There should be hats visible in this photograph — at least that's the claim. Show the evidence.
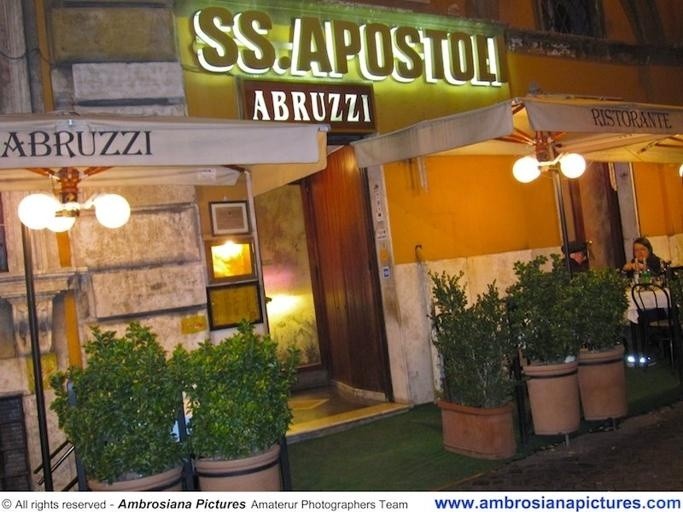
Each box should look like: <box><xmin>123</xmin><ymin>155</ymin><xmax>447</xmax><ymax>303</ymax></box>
<box><xmin>561</xmin><ymin>241</ymin><xmax>587</xmax><ymax>254</ymax></box>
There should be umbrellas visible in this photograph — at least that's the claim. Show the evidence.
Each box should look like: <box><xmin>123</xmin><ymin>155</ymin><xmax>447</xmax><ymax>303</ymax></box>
<box><xmin>0</xmin><ymin>113</ymin><xmax>331</xmax><ymax>368</ymax></box>
<box><xmin>351</xmin><ymin>92</ymin><xmax>683</xmax><ymax>278</ymax></box>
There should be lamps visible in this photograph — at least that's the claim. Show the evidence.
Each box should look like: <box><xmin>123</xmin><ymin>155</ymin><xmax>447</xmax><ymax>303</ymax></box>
<box><xmin>18</xmin><ymin>167</ymin><xmax>131</xmax><ymax>233</ymax></box>
<box><xmin>511</xmin><ymin>130</ymin><xmax>585</xmax><ymax>183</ymax></box>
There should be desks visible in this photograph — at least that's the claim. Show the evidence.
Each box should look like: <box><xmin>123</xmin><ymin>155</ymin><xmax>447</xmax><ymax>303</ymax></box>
<box><xmin>625</xmin><ymin>287</ymin><xmax>671</xmax><ymax>368</ymax></box>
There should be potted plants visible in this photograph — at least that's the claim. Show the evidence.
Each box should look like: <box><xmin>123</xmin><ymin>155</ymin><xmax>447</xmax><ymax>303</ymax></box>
<box><xmin>428</xmin><ymin>271</ymin><xmax>518</xmax><ymax>461</ymax></box>
<box><xmin>185</xmin><ymin>319</ymin><xmax>301</xmax><ymax>492</ymax></box>
<box><xmin>507</xmin><ymin>253</ymin><xmax>580</xmax><ymax>436</ymax></box>
<box><xmin>572</xmin><ymin>269</ymin><xmax>630</xmax><ymax>420</ymax></box>
<box><xmin>49</xmin><ymin>318</ymin><xmax>195</xmax><ymax>491</ymax></box>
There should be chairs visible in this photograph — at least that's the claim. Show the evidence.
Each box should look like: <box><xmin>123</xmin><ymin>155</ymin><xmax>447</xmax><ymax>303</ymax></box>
<box><xmin>631</xmin><ymin>283</ymin><xmax>674</xmax><ymax>372</ymax></box>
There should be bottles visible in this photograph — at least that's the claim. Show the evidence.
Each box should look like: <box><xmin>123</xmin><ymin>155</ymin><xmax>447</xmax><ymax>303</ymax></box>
<box><xmin>641</xmin><ymin>257</ymin><xmax>649</xmax><ymax>284</ymax></box>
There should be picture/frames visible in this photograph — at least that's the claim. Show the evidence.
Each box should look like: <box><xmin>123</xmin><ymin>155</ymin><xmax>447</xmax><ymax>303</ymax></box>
<box><xmin>203</xmin><ymin>236</ymin><xmax>258</xmax><ymax>285</ymax></box>
<box><xmin>206</xmin><ymin>280</ymin><xmax>264</xmax><ymax>331</ymax></box>
<box><xmin>208</xmin><ymin>199</ymin><xmax>251</xmax><ymax>237</ymax></box>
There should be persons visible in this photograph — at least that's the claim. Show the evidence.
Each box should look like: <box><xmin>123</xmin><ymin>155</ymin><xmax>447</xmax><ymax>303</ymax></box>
<box><xmin>559</xmin><ymin>241</ymin><xmax>588</xmax><ymax>276</ymax></box>
<box><xmin>619</xmin><ymin>236</ymin><xmax>663</xmax><ymax>279</ymax></box>
